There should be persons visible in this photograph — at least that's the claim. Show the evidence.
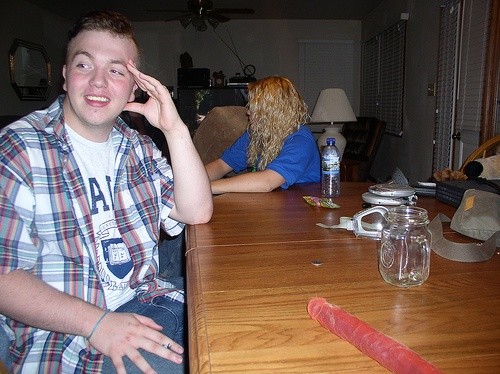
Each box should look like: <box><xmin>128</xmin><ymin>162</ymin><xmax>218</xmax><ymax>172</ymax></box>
<box><xmin>0</xmin><ymin>10</ymin><xmax>213</xmax><ymax>374</ymax></box>
<box><xmin>205</xmin><ymin>75</ymin><xmax>321</xmax><ymax>194</ymax></box>
<box><xmin>119</xmin><ymin>86</ymin><xmax>185</xmax><ymax>291</ymax></box>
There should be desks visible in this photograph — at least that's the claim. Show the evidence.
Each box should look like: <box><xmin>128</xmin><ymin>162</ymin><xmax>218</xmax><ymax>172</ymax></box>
<box><xmin>185</xmin><ymin>182</ymin><xmax>500</xmax><ymax>374</ymax></box>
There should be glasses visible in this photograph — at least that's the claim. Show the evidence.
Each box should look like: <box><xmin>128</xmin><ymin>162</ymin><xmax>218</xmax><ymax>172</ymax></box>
<box><xmin>353</xmin><ymin>205</ymin><xmax>389</xmax><ymax>240</ymax></box>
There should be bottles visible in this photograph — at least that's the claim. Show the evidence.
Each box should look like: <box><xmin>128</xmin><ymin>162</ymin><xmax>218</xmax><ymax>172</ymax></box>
<box><xmin>322</xmin><ymin>138</ymin><xmax>340</xmax><ymax>198</ymax></box>
<box><xmin>378</xmin><ymin>207</ymin><xmax>432</xmax><ymax>288</ymax></box>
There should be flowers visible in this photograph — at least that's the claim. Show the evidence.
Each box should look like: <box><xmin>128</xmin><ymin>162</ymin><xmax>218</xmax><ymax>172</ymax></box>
<box><xmin>193</xmin><ymin>89</ymin><xmax>210</xmax><ymax>110</ymax></box>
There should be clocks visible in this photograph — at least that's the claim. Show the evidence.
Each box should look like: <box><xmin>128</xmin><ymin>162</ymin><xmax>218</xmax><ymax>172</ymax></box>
<box><xmin>213</xmin><ymin>71</ymin><xmax>226</xmax><ymax>86</ymax></box>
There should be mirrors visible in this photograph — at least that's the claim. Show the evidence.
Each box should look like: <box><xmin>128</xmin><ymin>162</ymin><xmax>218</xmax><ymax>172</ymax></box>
<box><xmin>8</xmin><ymin>39</ymin><xmax>53</xmax><ymax>101</ymax></box>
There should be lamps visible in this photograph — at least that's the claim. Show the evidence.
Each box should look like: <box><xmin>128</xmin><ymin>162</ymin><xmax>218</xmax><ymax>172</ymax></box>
<box><xmin>309</xmin><ymin>88</ymin><xmax>358</xmax><ymax>162</ymax></box>
<box><xmin>180</xmin><ymin>8</ymin><xmax>219</xmax><ymax>31</ymax></box>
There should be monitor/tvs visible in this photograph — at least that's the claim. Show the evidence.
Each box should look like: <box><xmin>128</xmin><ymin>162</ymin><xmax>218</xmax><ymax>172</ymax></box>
<box><xmin>174</xmin><ymin>87</ymin><xmax>249</xmax><ymax>133</ymax></box>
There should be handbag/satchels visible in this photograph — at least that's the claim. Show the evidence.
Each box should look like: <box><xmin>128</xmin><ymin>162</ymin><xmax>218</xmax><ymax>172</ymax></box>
<box><xmin>449</xmin><ymin>188</ymin><xmax>500</xmax><ymax>241</ymax></box>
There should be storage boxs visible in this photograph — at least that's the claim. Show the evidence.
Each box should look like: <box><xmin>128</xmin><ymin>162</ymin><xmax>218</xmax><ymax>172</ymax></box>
<box><xmin>177</xmin><ymin>68</ymin><xmax>210</xmax><ymax>88</ymax></box>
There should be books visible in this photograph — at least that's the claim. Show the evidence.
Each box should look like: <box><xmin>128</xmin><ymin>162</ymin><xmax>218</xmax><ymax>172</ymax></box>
<box><xmin>414</xmin><ymin>179</ymin><xmax>498</xmax><ymax>209</ymax></box>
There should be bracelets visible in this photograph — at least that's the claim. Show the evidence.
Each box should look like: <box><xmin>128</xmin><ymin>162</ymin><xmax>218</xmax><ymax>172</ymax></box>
<box><xmin>86</xmin><ymin>309</ymin><xmax>110</xmax><ymax>340</ymax></box>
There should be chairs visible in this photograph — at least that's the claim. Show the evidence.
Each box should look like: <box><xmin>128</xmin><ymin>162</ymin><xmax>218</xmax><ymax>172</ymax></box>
<box><xmin>342</xmin><ymin>117</ymin><xmax>387</xmax><ymax>174</ymax></box>
<box><xmin>192</xmin><ymin>106</ymin><xmax>254</xmax><ymax>178</ymax></box>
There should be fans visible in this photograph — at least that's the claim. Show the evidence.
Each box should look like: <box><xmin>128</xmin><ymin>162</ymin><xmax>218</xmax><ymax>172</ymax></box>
<box><xmin>144</xmin><ymin>0</ymin><xmax>255</xmax><ymax>22</ymax></box>
